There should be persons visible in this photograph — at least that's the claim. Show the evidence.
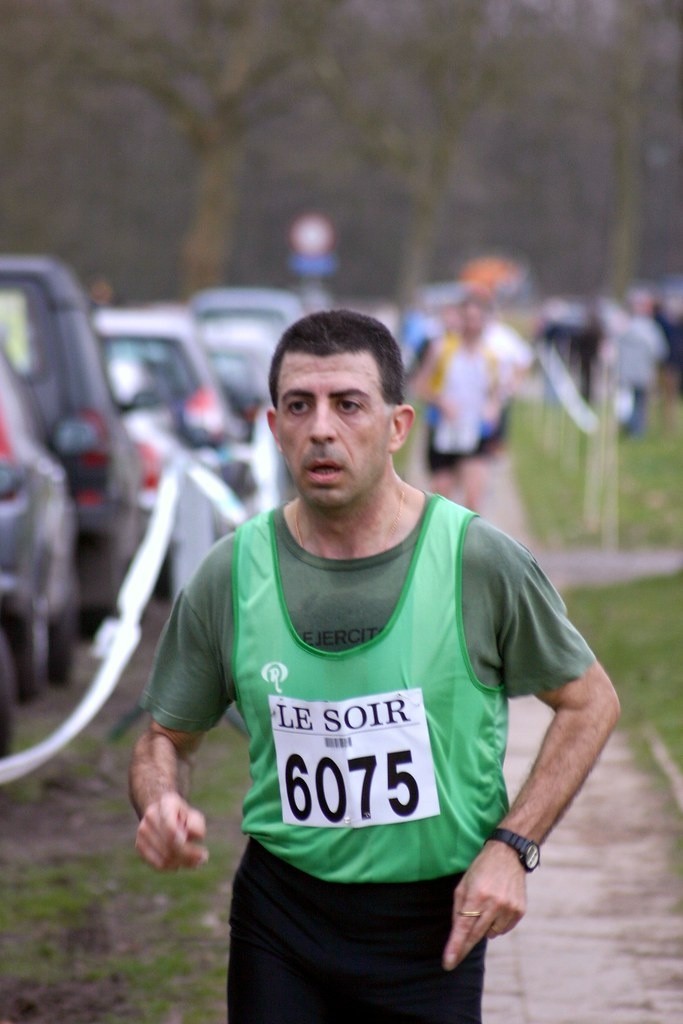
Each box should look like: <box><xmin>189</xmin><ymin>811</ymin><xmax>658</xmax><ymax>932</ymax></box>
<box><xmin>540</xmin><ymin>285</ymin><xmax>576</xmax><ymax>403</ymax></box>
<box><xmin>407</xmin><ymin>292</ymin><xmax>533</xmax><ymax>510</ymax></box>
<box><xmin>128</xmin><ymin>308</ymin><xmax>622</xmax><ymax>1024</ymax></box>
<box><xmin>604</xmin><ymin>288</ymin><xmax>683</xmax><ymax>437</ymax></box>
<box><xmin>89</xmin><ymin>282</ymin><xmax>111</xmax><ymax>308</ymax></box>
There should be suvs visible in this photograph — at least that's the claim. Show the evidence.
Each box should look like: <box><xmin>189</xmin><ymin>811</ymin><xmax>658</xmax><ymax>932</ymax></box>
<box><xmin>1</xmin><ymin>249</ymin><xmax>150</xmax><ymax>630</ymax></box>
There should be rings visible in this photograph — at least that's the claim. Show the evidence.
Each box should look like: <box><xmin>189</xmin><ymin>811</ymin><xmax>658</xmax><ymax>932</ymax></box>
<box><xmin>457</xmin><ymin>910</ymin><xmax>481</xmax><ymax>917</ymax></box>
<box><xmin>491</xmin><ymin>924</ymin><xmax>504</xmax><ymax>933</ymax></box>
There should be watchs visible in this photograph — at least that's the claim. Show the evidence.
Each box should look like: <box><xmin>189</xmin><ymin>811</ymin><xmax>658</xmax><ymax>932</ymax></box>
<box><xmin>483</xmin><ymin>828</ymin><xmax>540</xmax><ymax>873</ymax></box>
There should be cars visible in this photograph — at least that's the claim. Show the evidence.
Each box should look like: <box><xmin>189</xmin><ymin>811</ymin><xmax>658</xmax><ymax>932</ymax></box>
<box><xmin>1</xmin><ymin>352</ymin><xmax>98</xmax><ymax>766</ymax></box>
<box><xmin>87</xmin><ymin>277</ymin><xmax>314</xmax><ymax>600</ymax></box>
